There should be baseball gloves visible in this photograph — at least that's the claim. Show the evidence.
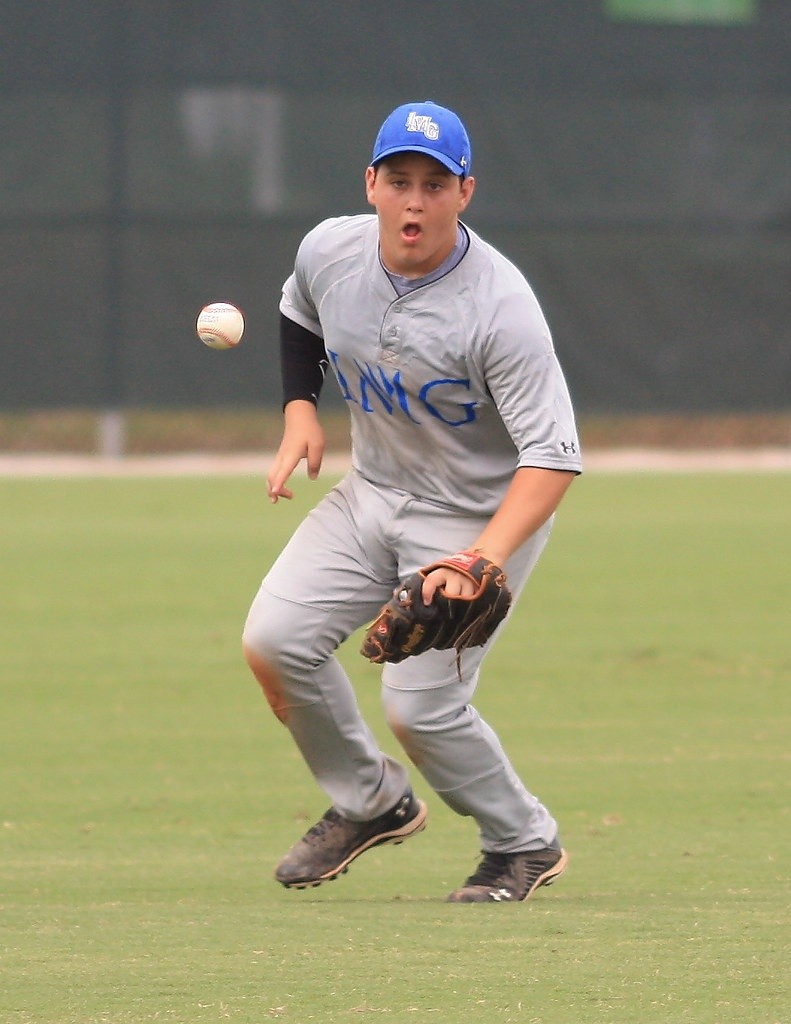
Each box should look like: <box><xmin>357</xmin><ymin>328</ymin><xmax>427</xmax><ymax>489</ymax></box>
<box><xmin>360</xmin><ymin>553</ymin><xmax>511</xmax><ymax>666</ymax></box>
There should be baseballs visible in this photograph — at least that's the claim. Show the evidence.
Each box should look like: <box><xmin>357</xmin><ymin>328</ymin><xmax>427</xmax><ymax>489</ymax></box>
<box><xmin>197</xmin><ymin>301</ymin><xmax>247</xmax><ymax>352</ymax></box>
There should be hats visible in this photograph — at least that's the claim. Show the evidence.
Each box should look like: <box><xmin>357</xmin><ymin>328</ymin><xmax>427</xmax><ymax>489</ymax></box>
<box><xmin>368</xmin><ymin>101</ymin><xmax>470</xmax><ymax>179</ymax></box>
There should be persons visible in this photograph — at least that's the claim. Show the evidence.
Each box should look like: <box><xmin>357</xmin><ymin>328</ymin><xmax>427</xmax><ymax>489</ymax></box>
<box><xmin>241</xmin><ymin>101</ymin><xmax>584</xmax><ymax>903</ymax></box>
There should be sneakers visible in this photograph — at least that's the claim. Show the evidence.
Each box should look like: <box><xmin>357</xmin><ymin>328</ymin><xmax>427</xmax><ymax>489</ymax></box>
<box><xmin>449</xmin><ymin>840</ymin><xmax>566</xmax><ymax>903</ymax></box>
<box><xmin>276</xmin><ymin>782</ymin><xmax>427</xmax><ymax>890</ymax></box>
